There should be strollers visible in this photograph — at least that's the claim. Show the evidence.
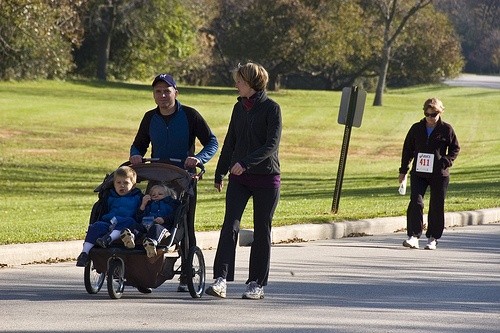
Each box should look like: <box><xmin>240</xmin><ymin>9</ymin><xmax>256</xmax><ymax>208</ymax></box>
<box><xmin>83</xmin><ymin>158</ymin><xmax>207</xmax><ymax>298</ymax></box>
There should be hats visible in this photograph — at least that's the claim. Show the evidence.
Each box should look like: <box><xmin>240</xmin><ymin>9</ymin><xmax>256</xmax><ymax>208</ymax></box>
<box><xmin>151</xmin><ymin>73</ymin><xmax>175</xmax><ymax>87</ymax></box>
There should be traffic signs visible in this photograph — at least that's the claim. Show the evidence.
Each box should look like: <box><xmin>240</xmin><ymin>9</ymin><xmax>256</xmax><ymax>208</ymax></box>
<box><xmin>331</xmin><ymin>86</ymin><xmax>367</xmax><ymax>216</ymax></box>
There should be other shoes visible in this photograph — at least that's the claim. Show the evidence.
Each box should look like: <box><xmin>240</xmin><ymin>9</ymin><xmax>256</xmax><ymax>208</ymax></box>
<box><xmin>75</xmin><ymin>252</ymin><xmax>89</xmax><ymax>267</ymax></box>
<box><xmin>96</xmin><ymin>235</ymin><xmax>111</xmax><ymax>248</ymax></box>
<box><xmin>120</xmin><ymin>227</ymin><xmax>136</xmax><ymax>248</ymax></box>
<box><xmin>143</xmin><ymin>238</ymin><xmax>157</xmax><ymax>258</ymax></box>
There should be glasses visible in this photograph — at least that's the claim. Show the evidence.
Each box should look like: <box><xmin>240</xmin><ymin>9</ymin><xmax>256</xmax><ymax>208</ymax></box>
<box><xmin>423</xmin><ymin>112</ymin><xmax>439</xmax><ymax>117</ymax></box>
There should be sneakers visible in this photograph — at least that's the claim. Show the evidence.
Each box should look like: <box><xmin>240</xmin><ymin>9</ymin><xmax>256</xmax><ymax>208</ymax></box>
<box><xmin>177</xmin><ymin>276</ymin><xmax>189</xmax><ymax>292</ymax></box>
<box><xmin>135</xmin><ymin>286</ymin><xmax>152</xmax><ymax>294</ymax></box>
<box><xmin>425</xmin><ymin>239</ymin><xmax>437</xmax><ymax>250</ymax></box>
<box><xmin>242</xmin><ymin>284</ymin><xmax>265</xmax><ymax>299</ymax></box>
<box><xmin>205</xmin><ymin>280</ymin><xmax>227</xmax><ymax>298</ymax></box>
<box><xmin>403</xmin><ymin>238</ymin><xmax>420</xmax><ymax>249</ymax></box>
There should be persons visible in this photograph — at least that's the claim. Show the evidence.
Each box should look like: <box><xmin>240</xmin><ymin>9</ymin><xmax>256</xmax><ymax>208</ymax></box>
<box><xmin>129</xmin><ymin>74</ymin><xmax>218</xmax><ymax>294</ymax></box>
<box><xmin>76</xmin><ymin>166</ymin><xmax>143</xmax><ymax>268</ymax></box>
<box><xmin>120</xmin><ymin>183</ymin><xmax>177</xmax><ymax>257</ymax></box>
<box><xmin>205</xmin><ymin>62</ymin><xmax>283</xmax><ymax>300</ymax></box>
<box><xmin>398</xmin><ymin>98</ymin><xmax>461</xmax><ymax>250</ymax></box>
